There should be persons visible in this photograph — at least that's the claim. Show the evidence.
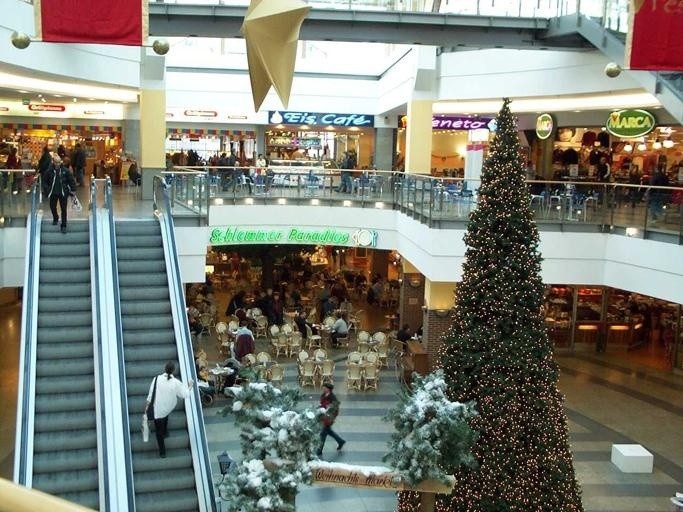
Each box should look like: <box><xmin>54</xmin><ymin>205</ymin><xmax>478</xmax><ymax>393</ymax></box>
<box><xmin>441</xmin><ymin>166</ymin><xmax>460</xmax><ymax>178</ymax></box>
<box><xmin>144</xmin><ymin>362</ymin><xmax>196</xmax><ymax>458</ymax></box>
<box><xmin>1</xmin><ymin>142</ymin><xmax>86</xmax><ymax>194</ymax></box>
<box><xmin>128</xmin><ymin>164</ymin><xmax>142</xmax><ymax>186</ymax></box>
<box><xmin>316</xmin><ymin>384</ymin><xmax>346</xmax><ymax>456</ymax></box>
<box><xmin>334</xmin><ymin>150</ymin><xmax>369</xmax><ymax>194</ymax></box>
<box><xmin>524</xmin><ymin>157</ymin><xmax>683</xmax><ymax>224</ymax></box>
<box><xmin>187</xmin><ymin>252</ymin><xmax>422</xmax><ymax>394</ymax></box>
<box><xmin>166</xmin><ymin>149</ymin><xmax>275</xmax><ymax>194</ymax></box>
<box><xmin>42</xmin><ymin>155</ymin><xmax>78</xmax><ymax>234</ymax></box>
<box><xmin>93</xmin><ymin>159</ymin><xmax>105</xmax><ymax>178</ymax></box>
<box><xmin>545</xmin><ymin>289</ymin><xmax>674</xmax><ymax>352</ymax></box>
<box><xmin>279</xmin><ymin>144</ymin><xmax>330</xmax><ymax>161</ymax></box>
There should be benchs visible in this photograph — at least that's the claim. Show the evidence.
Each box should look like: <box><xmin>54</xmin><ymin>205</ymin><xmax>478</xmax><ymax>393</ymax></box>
<box><xmin>611</xmin><ymin>444</ymin><xmax>654</xmax><ymax>473</ymax></box>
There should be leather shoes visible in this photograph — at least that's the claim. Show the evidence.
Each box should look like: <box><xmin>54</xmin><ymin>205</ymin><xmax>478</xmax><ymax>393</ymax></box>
<box><xmin>337</xmin><ymin>441</ymin><xmax>345</xmax><ymax>449</ymax></box>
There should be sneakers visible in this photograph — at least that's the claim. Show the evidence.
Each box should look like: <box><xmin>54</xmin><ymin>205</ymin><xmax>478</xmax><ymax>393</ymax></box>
<box><xmin>62</xmin><ymin>226</ymin><xmax>66</xmax><ymax>233</ymax></box>
<box><xmin>53</xmin><ymin>216</ymin><xmax>59</xmax><ymax>225</ymax></box>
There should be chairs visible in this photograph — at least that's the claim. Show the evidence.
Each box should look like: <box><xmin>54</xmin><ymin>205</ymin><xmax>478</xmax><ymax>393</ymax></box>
<box><xmin>189</xmin><ymin>268</ymin><xmax>408</xmax><ymax>392</ymax></box>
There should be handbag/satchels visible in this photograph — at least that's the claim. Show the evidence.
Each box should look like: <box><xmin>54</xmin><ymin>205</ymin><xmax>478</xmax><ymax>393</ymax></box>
<box><xmin>147</xmin><ymin>404</ymin><xmax>153</xmax><ymax>420</ymax></box>
<box><xmin>142</xmin><ymin>414</ymin><xmax>148</xmax><ymax>442</ymax></box>
